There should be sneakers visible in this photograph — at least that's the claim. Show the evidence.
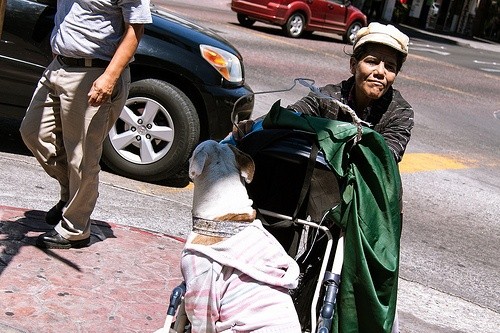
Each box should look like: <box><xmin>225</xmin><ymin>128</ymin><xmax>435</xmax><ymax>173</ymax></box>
<box><xmin>46</xmin><ymin>200</ymin><xmax>67</xmax><ymax>225</ymax></box>
<box><xmin>36</xmin><ymin>228</ymin><xmax>90</xmax><ymax>249</ymax></box>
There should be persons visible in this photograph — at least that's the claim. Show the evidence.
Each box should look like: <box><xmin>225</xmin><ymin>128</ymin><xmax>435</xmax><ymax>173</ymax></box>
<box><xmin>233</xmin><ymin>24</ymin><xmax>416</xmax><ymax>274</ymax></box>
<box><xmin>18</xmin><ymin>0</ymin><xmax>153</xmax><ymax>254</ymax></box>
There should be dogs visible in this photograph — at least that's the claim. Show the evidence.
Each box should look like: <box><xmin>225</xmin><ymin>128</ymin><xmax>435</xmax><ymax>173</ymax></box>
<box><xmin>179</xmin><ymin>139</ymin><xmax>304</xmax><ymax>333</ymax></box>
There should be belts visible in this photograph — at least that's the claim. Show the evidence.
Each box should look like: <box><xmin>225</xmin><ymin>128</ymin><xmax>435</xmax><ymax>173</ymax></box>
<box><xmin>59</xmin><ymin>55</ymin><xmax>111</xmax><ymax>68</ymax></box>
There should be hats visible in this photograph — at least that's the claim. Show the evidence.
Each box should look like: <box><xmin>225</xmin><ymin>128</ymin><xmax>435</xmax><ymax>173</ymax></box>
<box><xmin>353</xmin><ymin>22</ymin><xmax>409</xmax><ymax>63</ymax></box>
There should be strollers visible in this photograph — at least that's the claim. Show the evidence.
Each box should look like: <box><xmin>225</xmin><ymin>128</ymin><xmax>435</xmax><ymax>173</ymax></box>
<box><xmin>163</xmin><ymin>113</ymin><xmax>347</xmax><ymax>333</ymax></box>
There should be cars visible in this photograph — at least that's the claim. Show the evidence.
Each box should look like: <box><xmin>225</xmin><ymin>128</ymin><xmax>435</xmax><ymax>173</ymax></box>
<box><xmin>0</xmin><ymin>0</ymin><xmax>255</xmax><ymax>186</ymax></box>
<box><xmin>230</xmin><ymin>0</ymin><xmax>368</xmax><ymax>46</ymax></box>
